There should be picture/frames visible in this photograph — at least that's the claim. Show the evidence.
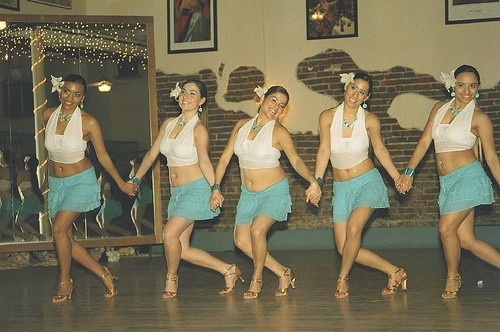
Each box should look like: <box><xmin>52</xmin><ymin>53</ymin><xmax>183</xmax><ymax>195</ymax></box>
<box><xmin>0</xmin><ymin>0</ymin><xmax>20</xmax><ymax>11</ymax></box>
<box><xmin>445</xmin><ymin>0</ymin><xmax>500</xmax><ymax>25</ymax></box>
<box><xmin>306</xmin><ymin>0</ymin><xmax>358</xmax><ymax>40</ymax></box>
<box><xmin>167</xmin><ymin>0</ymin><xmax>217</xmax><ymax>54</ymax></box>
<box><xmin>28</xmin><ymin>0</ymin><xmax>72</xmax><ymax>9</ymax></box>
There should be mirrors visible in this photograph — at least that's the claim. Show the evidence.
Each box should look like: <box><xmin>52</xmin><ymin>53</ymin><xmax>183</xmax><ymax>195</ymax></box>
<box><xmin>0</xmin><ymin>14</ymin><xmax>162</xmax><ymax>253</ymax></box>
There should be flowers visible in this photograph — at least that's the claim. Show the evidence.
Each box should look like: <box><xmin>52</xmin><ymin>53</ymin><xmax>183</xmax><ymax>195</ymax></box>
<box><xmin>170</xmin><ymin>81</ymin><xmax>182</xmax><ymax>100</ymax></box>
<box><xmin>254</xmin><ymin>85</ymin><xmax>269</xmax><ymax>97</ymax></box>
<box><xmin>50</xmin><ymin>75</ymin><xmax>65</xmax><ymax>92</ymax></box>
<box><xmin>440</xmin><ymin>69</ymin><xmax>456</xmax><ymax>90</ymax></box>
<box><xmin>339</xmin><ymin>72</ymin><xmax>355</xmax><ymax>90</ymax></box>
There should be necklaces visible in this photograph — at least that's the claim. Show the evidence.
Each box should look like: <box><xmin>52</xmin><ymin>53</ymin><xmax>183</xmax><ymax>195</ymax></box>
<box><xmin>342</xmin><ymin>114</ymin><xmax>358</xmax><ymax>128</ymax></box>
<box><xmin>252</xmin><ymin>120</ymin><xmax>263</xmax><ymax>131</ymax></box>
<box><xmin>58</xmin><ymin>107</ymin><xmax>72</xmax><ymax>122</ymax></box>
<box><xmin>177</xmin><ymin>116</ymin><xmax>188</xmax><ymax>127</ymax></box>
<box><xmin>449</xmin><ymin>99</ymin><xmax>463</xmax><ymax>116</ymax></box>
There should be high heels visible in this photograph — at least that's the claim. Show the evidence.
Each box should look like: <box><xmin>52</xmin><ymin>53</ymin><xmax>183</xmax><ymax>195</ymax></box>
<box><xmin>243</xmin><ymin>278</ymin><xmax>262</xmax><ymax>300</ymax></box>
<box><xmin>100</xmin><ymin>266</ymin><xmax>118</xmax><ymax>297</ymax></box>
<box><xmin>275</xmin><ymin>268</ymin><xmax>296</xmax><ymax>297</ymax></box>
<box><xmin>381</xmin><ymin>268</ymin><xmax>407</xmax><ymax>294</ymax></box>
<box><xmin>52</xmin><ymin>278</ymin><xmax>73</xmax><ymax>302</ymax></box>
<box><xmin>162</xmin><ymin>276</ymin><xmax>179</xmax><ymax>299</ymax></box>
<box><xmin>335</xmin><ymin>276</ymin><xmax>351</xmax><ymax>297</ymax></box>
<box><xmin>442</xmin><ymin>274</ymin><xmax>462</xmax><ymax>298</ymax></box>
<box><xmin>217</xmin><ymin>264</ymin><xmax>244</xmax><ymax>294</ymax></box>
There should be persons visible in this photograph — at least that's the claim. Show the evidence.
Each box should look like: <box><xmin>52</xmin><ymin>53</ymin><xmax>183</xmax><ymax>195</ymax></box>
<box><xmin>127</xmin><ymin>78</ymin><xmax>244</xmax><ymax>298</ymax></box>
<box><xmin>126</xmin><ymin>154</ymin><xmax>154</xmax><ymax>236</ymax></box>
<box><xmin>209</xmin><ymin>86</ymin><xmax>322</xmax><ymax>299</ymax></box>
<box><xmin>0</xmin><ymin>152</ymin><xmax>33</xmax><ymax>243</ymax></box>
<box><xmin>96</xmin><ymin>156</ymin><xmax>122</xmax><ymax>239</ymax></box>
<box><xmin>395</xmin><ymin>66</ymin><xmax>500</xmax><ymax>299</ymax></box>
<box><xmin>72</xmin><ymin>212</ymin><xmax>110</xmax><ymax>240</ymax></box>
<box><xmin>305</xmin><ymin>72</ymin><xmax>414</xmax><ymax>298</ymax></box>
<box><xmin>15</xmin><ymin>155</ymin><xmax>49</xmax><ymax>242</ymax></box>
<box><xmin>41</xmin><ymin>74</ymin><xmax>137</xmax><ymax>302</ymax></box>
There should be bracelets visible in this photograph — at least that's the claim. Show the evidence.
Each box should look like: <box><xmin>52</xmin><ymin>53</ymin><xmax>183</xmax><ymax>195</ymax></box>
<box><xmin>210</xmin><ymin>186</ymin><xmax>214</xmax><ymax>193</ymax></box>
<box><xmin>313</xmin><ymin>175</ymin><xmax>323</xmax><ymax>186</ymax></box>
<box><xmin>212</xmin><ymin>184</ymin><xmax>221</xmax><ymax>191</ymax></box>
<box><xmin>130</xmin><ymin>177</ymin><xmax>142</xmax><ymax>186</ymax></box>
<box><xmin>402</xmin><ymin>168</ymin><xmax>415</xmax><ymax>177</ymax></box>
